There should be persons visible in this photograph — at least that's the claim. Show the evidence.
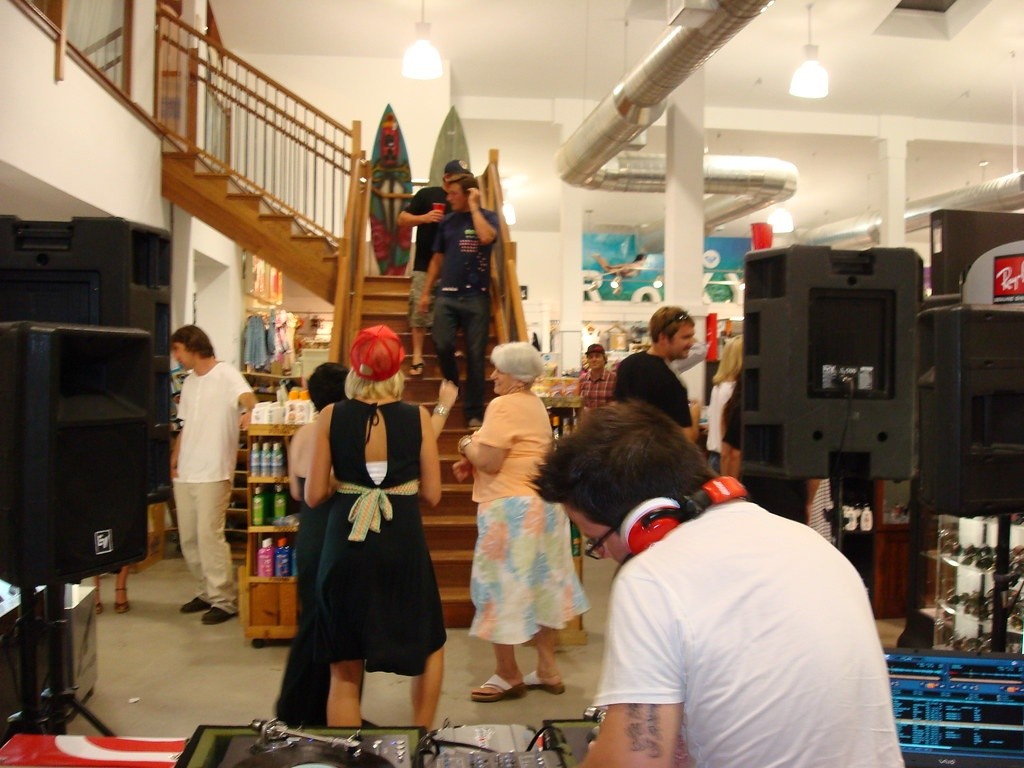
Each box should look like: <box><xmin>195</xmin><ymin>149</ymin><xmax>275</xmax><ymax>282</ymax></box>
<box><xmin>531</xmin><ymin>399</ymin><xmax>910</xmax><ymax>768</ymax></box>
<box><xmin>271</xmin><ymin>159</ymin><xmax>834</xmax><ymax>727</ymax></box>
<box><xmin>95</xmin><ymin>324</ymin><xmax>261</xmax><ymax>625</ymax></box>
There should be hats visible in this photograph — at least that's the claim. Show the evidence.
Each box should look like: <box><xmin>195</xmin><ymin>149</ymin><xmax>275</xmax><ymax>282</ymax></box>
<box><xmin>585</xmin><ymin>344</ymin><xmax>604</xmax><ymax>355</ymax></box>
<box><xmin>444</xmin><ymin>160</ymin><xmax>473</xmax><ymax>175</ymax></box>
<box><xmin>350</xmin><ymin>324</ymin><xmax>405</xmax><ymax>380</ymax></box>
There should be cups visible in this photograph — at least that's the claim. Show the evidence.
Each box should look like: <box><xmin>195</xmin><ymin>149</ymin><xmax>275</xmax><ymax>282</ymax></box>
<box><xmin>292</xmin><ymin>362</ymin><xmax>301</xmax><ymax>377</ymax></box>
<box><xmin>751</xmin><ymin>223</ymin><xmax>773</xmax><ymax>250</ymax></box>
<box><xmin>433</xmin><ymin>203</ymin><xmax>445</xmax><ymax>222</ymax></box>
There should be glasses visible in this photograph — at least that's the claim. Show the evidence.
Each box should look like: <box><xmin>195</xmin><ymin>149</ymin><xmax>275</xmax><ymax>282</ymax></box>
<box><xmin>658</xmin><ymin>312</ymin><xmax>689</xmax><ymax>333</ymax></box>
<box><xmin>584</xmin><ymin>515</ymin><xmax>627</xmax><ymax>560</ymax></box>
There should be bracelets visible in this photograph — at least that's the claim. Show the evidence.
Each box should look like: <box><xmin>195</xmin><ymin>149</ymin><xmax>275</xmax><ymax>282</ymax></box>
<box><xmin>460</xmin><ymin>440</ymin><xmax>473</xmax><ymax>455</ymax></box>
<box><xmin>457</xmin><ymin>435</ymin><xmax>470</xmax><ymax>446</ymax></box>
<box><xmin>434</xmin><ymin>404</ymin><xmax>449</xmax><ymax>415</ymax></box>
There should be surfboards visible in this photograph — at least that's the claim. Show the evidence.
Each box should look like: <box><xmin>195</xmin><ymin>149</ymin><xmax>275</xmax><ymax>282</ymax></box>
<box><xmin>369</xmin><ymin>104</ymin><xmax>412</xmax><ymax>276</ymax></box>
<box><xmin>429</xmin><ymin>106</ymin><xmax>470</xmax><ymax>188</ymax></box>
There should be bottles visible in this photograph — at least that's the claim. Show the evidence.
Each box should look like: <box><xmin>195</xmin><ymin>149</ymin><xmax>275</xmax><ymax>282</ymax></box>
<box><xmin>257</xmin><ymin>536</ymin><xmax>275</xmax><ymax>577</ymax></box>
<box><xmin>251</xmin><ymin>401</ymin><xmax>271</xmax><ymax>424</ymax></box>
<box><xmin>275</xmin><ymin>537</ymin><xmax>291</xmax><ymax>577</ymax></box>
<box><xmin>271</xmin><ymin>403</ymin><xmax>285</xmax><ymax>424</ymax></box>
<box><xmin>286</xmin><ymin>388</ymin><xmax>314</xmax><ymax>425</ymax></box>
<box><xmin>292</xmin><ymin>548</ymin><xmax>298</xmax><ymax>576</ymax></box>
<box><xmin>552</xmin><ymin>417</ymin><xmax>572</xmax><ymax>449</ymax></box>
<box><xmin>270</xmin><ymin>484</ymin><xmax>287</xmax><ymax>526</ymax></box>
<box><xmin>251</xmin><ymin>442</ymin><xmax>283</xmax><ymax>466</ymax></box>
<box><xmin>252</xmin><ymin>486</ymin><xmax>270</xmax><ymax>525</ymax></box>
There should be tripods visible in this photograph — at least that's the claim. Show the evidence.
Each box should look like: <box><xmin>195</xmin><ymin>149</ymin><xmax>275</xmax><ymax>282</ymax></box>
<box><xmin>0</xmin><ymin>585</ymin><xmax>115</xmax><ymax>748</ymax></box>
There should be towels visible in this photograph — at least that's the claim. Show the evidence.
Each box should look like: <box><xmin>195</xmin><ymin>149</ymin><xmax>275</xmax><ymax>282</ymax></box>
<box><xmin>244</xmin><ymin>308</ymin><xmax>293</xmax><ymax>367</ymax></box>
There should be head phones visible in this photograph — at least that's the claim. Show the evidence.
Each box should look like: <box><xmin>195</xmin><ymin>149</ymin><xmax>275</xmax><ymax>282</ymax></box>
<box><xmin>621</xmin><ymin>475</ymin><xmax>748</xmax><ymax>555</ymax></box>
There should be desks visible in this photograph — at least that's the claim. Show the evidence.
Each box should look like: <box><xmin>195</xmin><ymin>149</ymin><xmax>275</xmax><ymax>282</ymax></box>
<box><xmin>0</xmin><ymin>733</ymin><xmax>188</xmax><ymax>768</ymax></box>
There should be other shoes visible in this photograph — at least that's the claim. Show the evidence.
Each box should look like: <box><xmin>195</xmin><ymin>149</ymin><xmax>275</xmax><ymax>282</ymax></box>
<box><xmin>181</xmin><ymin>596</ymin><xmax>211</xmax><ymax>612</ymax></box>
<box><xmin>202</xmin><ymin>607</ymin><xmax>236</xmax><ymax>624</ymax></box>
<box><xmin>95</xmin><ymin>602</ymin><xmax>102</xmax><ymax>615</ymax></box>
<box><xmin>467</xmin><ymin>418</ymin><xmax>481</xmax><ymax>431</ymax></box>
<box><xmin>115</xmin><ymin>588</ymin><xmax>129</xmax><ymax>613</ymax></box>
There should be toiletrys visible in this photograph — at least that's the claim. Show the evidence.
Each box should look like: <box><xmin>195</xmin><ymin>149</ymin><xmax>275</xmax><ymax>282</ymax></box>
<box><xmin>271</xmin><ymin>483</ymin><xmax>289</xmax><ymax>519</ymax></box>
<box><xmin>250</xmin><ymin>389</ymin><xmax>316</xmax><ymax>425</ymax></box>
<box><xmin>253</xmin><ymin>484</ymin><xmax>268</xmax><ymax>525</ymax></box>
<box><xmin>257</xmin><ymin>538</ymin><xmax>299</xmax><ymax>578</ymax></box>
<box><xmin>249</xmin><ymin>442</ymin><xmax>287</xmax><ymax>477</ymax></box>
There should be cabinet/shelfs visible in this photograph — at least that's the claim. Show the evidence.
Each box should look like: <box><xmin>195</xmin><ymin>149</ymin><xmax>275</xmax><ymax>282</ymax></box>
<box><xmin>917</xmin><ymin>515</ymin><xmax>1024</xmax><ymax>651</ymax></box>
<box><xmin>225</xmin><ymin>422</ymin><xmax>301</xmax><ymax>641</ymax></box>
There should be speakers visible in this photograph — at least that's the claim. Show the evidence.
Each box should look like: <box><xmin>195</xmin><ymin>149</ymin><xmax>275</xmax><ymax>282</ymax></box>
<box><xmin>0</xmin><ymin>214</ymin><xmax>172</xmax><ymax>590</ymax></box>
<box><xmin>737</xmin><ymin>243</ymin><xmax>1024</xmax><ymax>519</ymax></box>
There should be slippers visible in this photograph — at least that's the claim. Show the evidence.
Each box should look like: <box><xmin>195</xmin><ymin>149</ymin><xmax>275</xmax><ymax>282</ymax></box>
<box><xmin>522</xmin><ymin>670</ymin><xmax>565</xmax><ymax>693</ymax></box>
<box><xmin>409</xmin><ymin>364</ymin><xmax>423</xmax><ymax>377</ymax></box>
<box><xmin>472</xmin><ymin>671</ymin><xmax>526</xmax><ymax>703</ymax></box>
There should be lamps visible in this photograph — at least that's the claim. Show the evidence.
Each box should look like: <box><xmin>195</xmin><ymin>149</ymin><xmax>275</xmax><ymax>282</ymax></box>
<box><xmin>767</xmin><ymin>202</ymin><xmax>794</xmax><ymax>233</ymax></box>
<box><xmin>401</xmin><ymin>0</ymin><xmax>443</xmax><ymax>79</ymax></box>
<box><xmin>789</xmin><ymin>4</ymin><xmax>828</xmax><ymax>98</ymax></box>
